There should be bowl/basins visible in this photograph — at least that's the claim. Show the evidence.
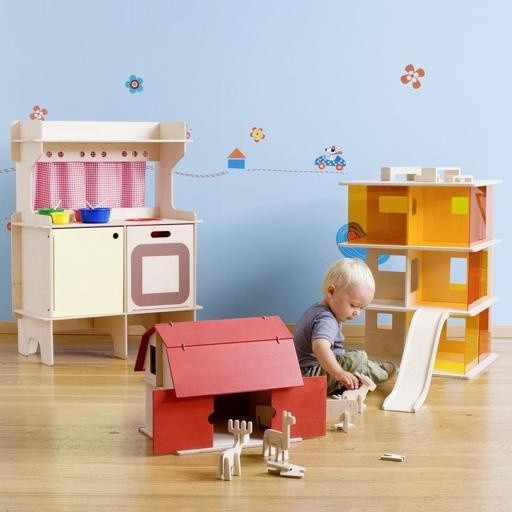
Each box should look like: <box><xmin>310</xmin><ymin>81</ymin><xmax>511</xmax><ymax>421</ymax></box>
<box><xmin>38</xmin><ymin>207</ymin><xmax>110</xmax><ymax>224</ymax></box>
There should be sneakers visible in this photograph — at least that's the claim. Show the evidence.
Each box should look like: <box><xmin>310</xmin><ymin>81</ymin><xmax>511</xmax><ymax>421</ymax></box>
<box><xmin>378</xmin><ymin>361</ymin><xmax>396</xmax><ymax>378</ymax></box>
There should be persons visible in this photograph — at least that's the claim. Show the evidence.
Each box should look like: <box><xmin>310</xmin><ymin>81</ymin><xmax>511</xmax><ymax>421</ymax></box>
<box><xmin>290</xmin><ymin>257</ymin><xmax>396</xmax><ymax>397</ymax></box>
